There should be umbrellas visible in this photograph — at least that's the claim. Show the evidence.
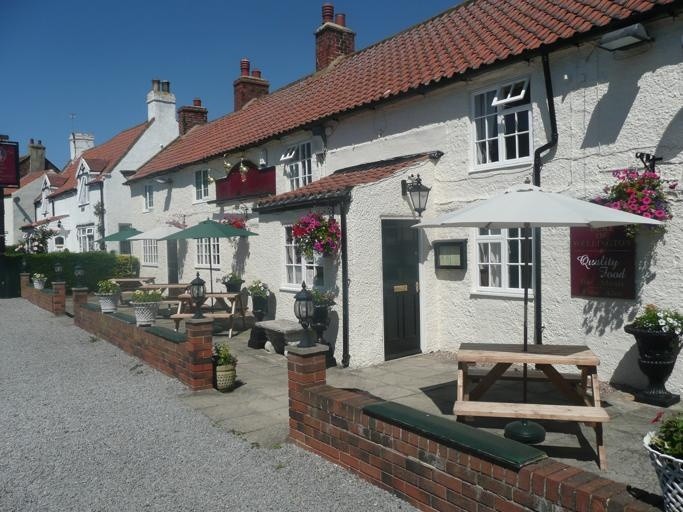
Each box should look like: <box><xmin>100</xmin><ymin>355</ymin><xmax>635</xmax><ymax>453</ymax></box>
<box><xmin>126</xmin><ymin>223</ymin><xmax>185</xmax><ymax>283</ymax></box>
<box><xmin>412</xmin><ymin>179</ymin><xmax>666</xmax><ymax>354</ymax></box>
<box><xmin>158</xmin><ymin>217</ymin><xmax>259</xmax><ymax>307</ymax></box>
<box><xmin>92</xmin><ymin>227</ymin><xmax>146</xmax><ymax>274</ymax></box>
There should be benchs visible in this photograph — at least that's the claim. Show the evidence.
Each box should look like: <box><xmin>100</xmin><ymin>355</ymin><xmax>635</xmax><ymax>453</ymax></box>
<box><xmin>466</xmin><ymin>367</ymin><xmax>584</xmax><ymax>385</ymax></box>
<box><xmin>453</xmin><ymin>399</ymin><xmax>611</xmax><ymax>428</ymax></box>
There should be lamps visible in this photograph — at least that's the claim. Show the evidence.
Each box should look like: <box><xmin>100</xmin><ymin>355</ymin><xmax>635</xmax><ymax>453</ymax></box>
<box><xmin>401</xmin><ymin>173</ymin><xmax>432</xmax><ymax>217</ymax></box>
<box><xmin>152</xmin><ymin>177</ymin><xmax>172</xmax><ymax>184</ymax></box>
<box><xmin>598</xmin><ymin>23</ymin><xmax>656</xmax><ymax>52</ymax></box>
<box><xmin>222</xmin><ymin>150</ymin><xmax>249</xmax><ymax>176</ymax></box>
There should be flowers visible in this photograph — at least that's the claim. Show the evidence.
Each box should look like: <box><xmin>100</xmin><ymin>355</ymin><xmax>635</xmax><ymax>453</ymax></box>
<box><xmin>644</xmin><ymin>408</ymin><xmax>683</xmax><ymax>462</ymax></box>
<box><xmin>291</xmin><ymin>212</ymin><xmax>340</xmax><ymax>259</ymax></box>
<box><xmin>211</xmin><ymin>342</ymin><xmax>238</xmax><ymax>365</ymax></box>
<box><xmin>247</xmin><ymin>280</ymin><xmax>270</xmax><ymax>297</ymax></box>
<box><xmin>33</xmin><ymin>272</ymin><xmax>45</xmax><ymax>281</ymax></box>
<box><xmin>95</xmin><ymin>278</ymin><xmax>121</xmax><ymax>294</ymax></box>
<box><xmin>308</xmin><ymin>287</ymin><xmax>337</xmax><ymax>306</ymax></box>
<box><xmin>624</xmin><ymin>304</ymin><xmax>683</xmax><ymax>354</ymax></box>
<box><xmin>589</xmin><ymin>166</ymin><xmax>677</xmax><ymax>238</ymax></box>
<box><xmin>222</xmin><ymin>212</ymin><xmax>247</xmax><ymax>231</ymax></box>
<box><xmin>132</xmin><ymin>288</ymin><xmax>162</xmax><ymax>302</ymax></box>
<box><xmin>221</xmin><ymin>272</ymin><xmax>242</xmax><ymax>284</ymax></box>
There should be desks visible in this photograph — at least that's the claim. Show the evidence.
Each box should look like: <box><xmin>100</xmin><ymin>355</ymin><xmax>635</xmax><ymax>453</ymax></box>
<box><xmin>452</xmin><ymin>342</ymin><xmax>604</xmax><ymax>471</ymax></box>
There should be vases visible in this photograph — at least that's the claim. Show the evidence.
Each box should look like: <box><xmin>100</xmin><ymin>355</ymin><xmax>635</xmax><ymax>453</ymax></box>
<box><xmin>129</xmin><ymin>301</ymin><xmax>159</xmax><ymax>327</ymax></box>
<box><xmin>222</xmin><ymin>280</ymin><xmax>245</xmax><ymax>293</ymax></box>
<box><xmin>31</xmin><ymin>277</ymin><xmax>48</xmax><ymax>289</ymax></box>
<box><xmin>624</xmin><ymin>324</ymin><xmax>683</xmax><ymax>408</ymax></box>
<box><xmin>248</xmin><ymin>295</ymin><xmax>268</xmax><ymax>349</ymax></box>
<box><xmin>214</xmin><ymin>362</ymin><xmax>238</xmax><ymax>392</ymax></box>
<box><xmin>310</xmin><ymin>305</ymin><xmax>336</xmax><ymax>368</ymax></box>
<box><xmin>644</xmin><ymin>431</ymin><xmax>683</xmax><ymax>512</ymax></box>
<box><xmin>95</xmin><ymin>293</ymin><xmax>120</xmax><ymax>314</ymax></box>
